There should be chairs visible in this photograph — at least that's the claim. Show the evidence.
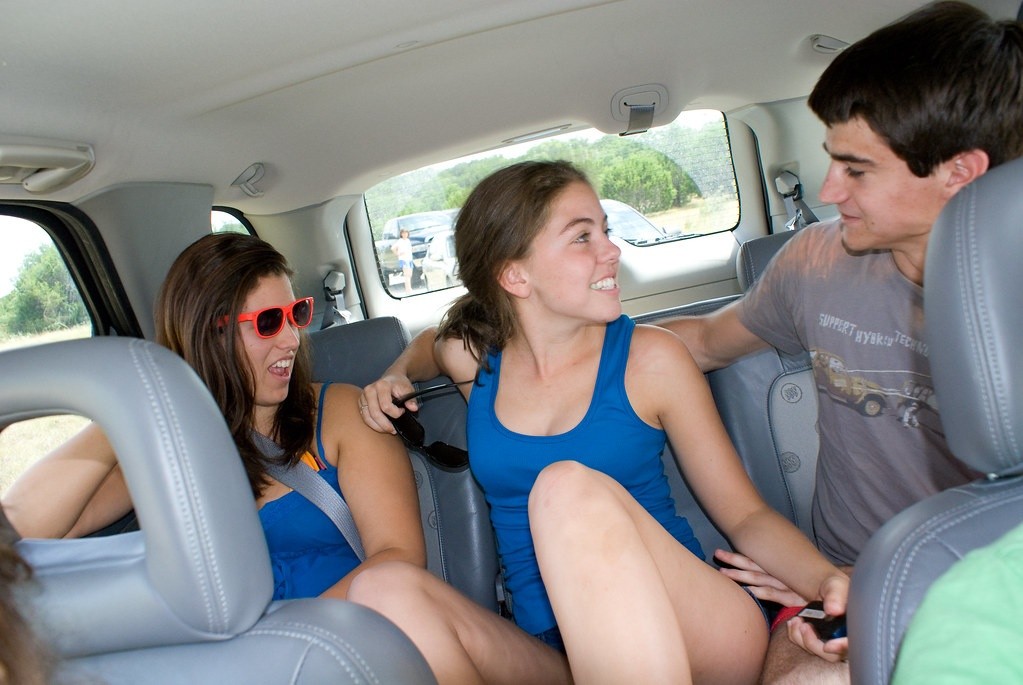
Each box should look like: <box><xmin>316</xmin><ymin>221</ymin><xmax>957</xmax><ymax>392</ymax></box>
<box><xmin>0</xmin><ymin>335</ymin><xmax>441</xmax><ymax>685</ymax></box>
<box><xmin>846</xmin><ymin>160</ymin><xmax>1023</xmax><ymax>685</ymax></box>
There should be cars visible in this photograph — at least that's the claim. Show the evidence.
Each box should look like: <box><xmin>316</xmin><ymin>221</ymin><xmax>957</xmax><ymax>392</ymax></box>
<box><xmin>445</xmin><ymin>208</ymin><xmax>463</xmax><ymax>219</ymax></box>
<box><xmin>423</xmin><ymin>227</ymin><xmax>468</xmax><ymax>292</ymax></box>
<box><xmin>599</xmin><ymin>198</ymin><xmax>705</xmax><ymax>244</ymax></box>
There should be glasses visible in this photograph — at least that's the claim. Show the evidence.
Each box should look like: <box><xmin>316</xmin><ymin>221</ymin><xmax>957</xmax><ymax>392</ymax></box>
<box><xmin>384</xmin><ymin>379</ymin><xmax>475</xmax><ymax>468</ymax></box>
<box><xmin>208</xmin><ymin>297</ymin><xmax>314</xmax><ymax>338</ymax></box>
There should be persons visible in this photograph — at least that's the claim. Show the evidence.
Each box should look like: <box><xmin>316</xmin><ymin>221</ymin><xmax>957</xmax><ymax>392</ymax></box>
<box><xmin>348</xmin><ymin>158</ymin><xmax>851</xmax><ymax>685</ymax></box>
<box><xmin>391</xmin><ymin>228</ymin><xmax>414</xmax><ymax>291</ymax></box>
<box><xmin>644</xmin><ymin>1</ymin><xmax>1022</xmax><ymax>685</ymax></box>
<box><xmin>2</xmin><ymin>230</ymin><xmax>430</xmax><ymax>598</ymax></box>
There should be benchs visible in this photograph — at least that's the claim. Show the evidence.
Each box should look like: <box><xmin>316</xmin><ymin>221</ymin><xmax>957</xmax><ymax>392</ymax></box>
<box><xmin>303</xmin><ymin>228</ymin><xmax>824</xmax><ymax>613</ymax></box>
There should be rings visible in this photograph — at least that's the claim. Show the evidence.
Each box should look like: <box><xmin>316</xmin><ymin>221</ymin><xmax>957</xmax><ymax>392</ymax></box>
<box><xmin>360</xmin><ymin>405</ymin><xmax>368</xmax><ymax>410</ymax></box>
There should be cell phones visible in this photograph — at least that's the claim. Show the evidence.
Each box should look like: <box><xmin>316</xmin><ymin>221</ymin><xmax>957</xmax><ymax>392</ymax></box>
<box><xmin>797</xmin><ymin>599</ymin><xmax>850</xmax><ymax>640</ymax></box>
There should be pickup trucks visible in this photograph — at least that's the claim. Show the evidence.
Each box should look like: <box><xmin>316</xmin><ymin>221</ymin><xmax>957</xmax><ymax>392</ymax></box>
<box><xmin>371</xmin><ymin>212</ymin><xmax>455</xmax><ymax>287</ymax></box>
